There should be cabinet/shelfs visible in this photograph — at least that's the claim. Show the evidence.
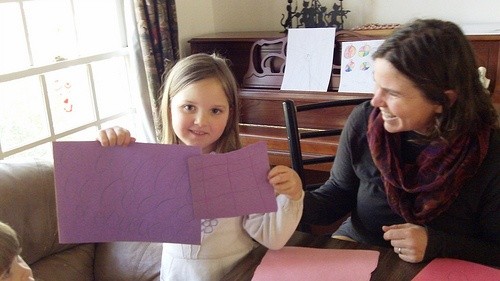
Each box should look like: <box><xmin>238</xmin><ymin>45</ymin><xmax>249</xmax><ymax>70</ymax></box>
<box><xmin>187</xmin><ymin>31</ymin><xmax>500</xmax><ymax>173</ymax></box>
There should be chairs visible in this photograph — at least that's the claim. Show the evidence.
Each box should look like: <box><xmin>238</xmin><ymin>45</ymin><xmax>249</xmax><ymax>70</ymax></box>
<box><xmin>284</xmin><ymin>98</ymin><xmax>372</xmax><ymax>232</ymax></box>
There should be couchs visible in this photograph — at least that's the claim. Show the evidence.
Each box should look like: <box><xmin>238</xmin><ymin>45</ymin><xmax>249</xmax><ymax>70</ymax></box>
<box><xmin>0</xmin><ymin>159</ymin><xmax>162</xmax><ymax>281</ymax></box>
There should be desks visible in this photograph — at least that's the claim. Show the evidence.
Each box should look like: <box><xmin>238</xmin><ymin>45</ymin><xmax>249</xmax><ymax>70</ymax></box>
<box><xmin>221</xmin><ymin>232</ymin><xmax>500</xmax><ymax>281</ymax></box>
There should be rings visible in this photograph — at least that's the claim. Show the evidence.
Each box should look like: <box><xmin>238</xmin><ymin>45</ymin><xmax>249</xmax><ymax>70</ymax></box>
<box><xmin>398</xmin><ymin>247</ymin><xmax>401</xmax><ymax>254</ymax></box>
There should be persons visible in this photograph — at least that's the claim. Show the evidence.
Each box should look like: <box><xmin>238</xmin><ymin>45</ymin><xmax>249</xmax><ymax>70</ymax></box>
<box><xmin>0</xmin><ymin>221</ymin><xmax>37</xmax><ymax>281</ymax></box>
<box><xmin>295</xmin><ymin>19</ymin><xmax>500</xmax><ymax>281</ymax></box>
<box><xmin>95</xmin><ymin>51</ymin><xmax>305</xmax><ymax>281</ymax></box>
